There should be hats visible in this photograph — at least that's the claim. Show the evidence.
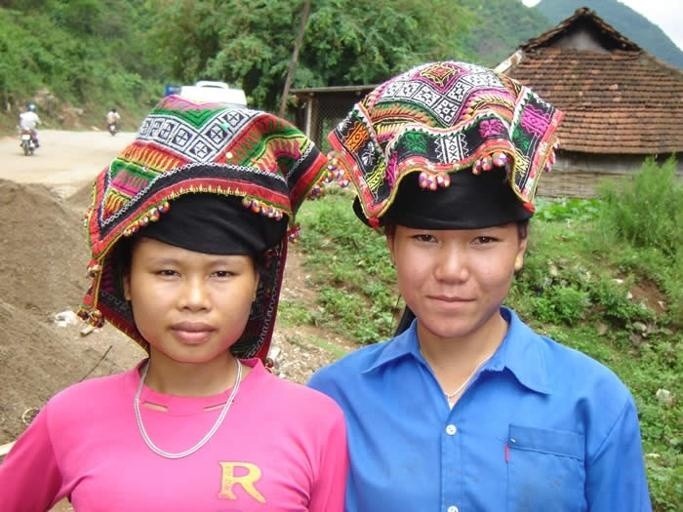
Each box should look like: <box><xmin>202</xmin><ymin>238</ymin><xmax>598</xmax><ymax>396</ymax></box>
<box><xmin>133</xmin><ymin>190</ymin><xmax>289</xmax><ymax>256</ymax></box>
<box><xmin>385</xmin><ymin>167</ymin><xmax>534</xmax><ymax>230</ymax></box>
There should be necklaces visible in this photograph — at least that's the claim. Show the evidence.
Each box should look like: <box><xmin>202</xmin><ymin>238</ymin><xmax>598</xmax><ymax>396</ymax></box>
<box><xmin>134</xmin><ymin>357</ymin><xmax>244</xmax><ymax>460</ymax></box>
<box><xmin>443</xmin><ymin>353</ymin><xmax>492</xmax><ymax>398</ymax></box>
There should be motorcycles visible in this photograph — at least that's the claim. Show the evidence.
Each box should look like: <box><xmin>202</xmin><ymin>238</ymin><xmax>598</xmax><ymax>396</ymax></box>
<box><xmin>19</xmin><ymin>127</ymin><xmax>38</xmax><ymax>156</ymax></box>
<box><xmin>107</xmin><ymin>123</ymin><xmax>119</xmax><ymax>135</ymax></box>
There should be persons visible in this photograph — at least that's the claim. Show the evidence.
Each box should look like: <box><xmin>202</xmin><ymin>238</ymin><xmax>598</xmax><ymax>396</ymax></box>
<box><xmin>18</xmin><ymin>103</ymin><xmax>41</xmax><ymax>150</ymax></box>
<box><xmin>306</xmin><ymin>59</ymin><xmax>653</xmax><ymax>512</ymax></box>
<box><xmin>107</xmin><ymin>108</ymin><xmax>121</xmax><ymax>134</ymax></box>
<box><xmin>0</xmin><ymin>91</ymin><xmax>351</xmax><ymax>512</ymax></box>
